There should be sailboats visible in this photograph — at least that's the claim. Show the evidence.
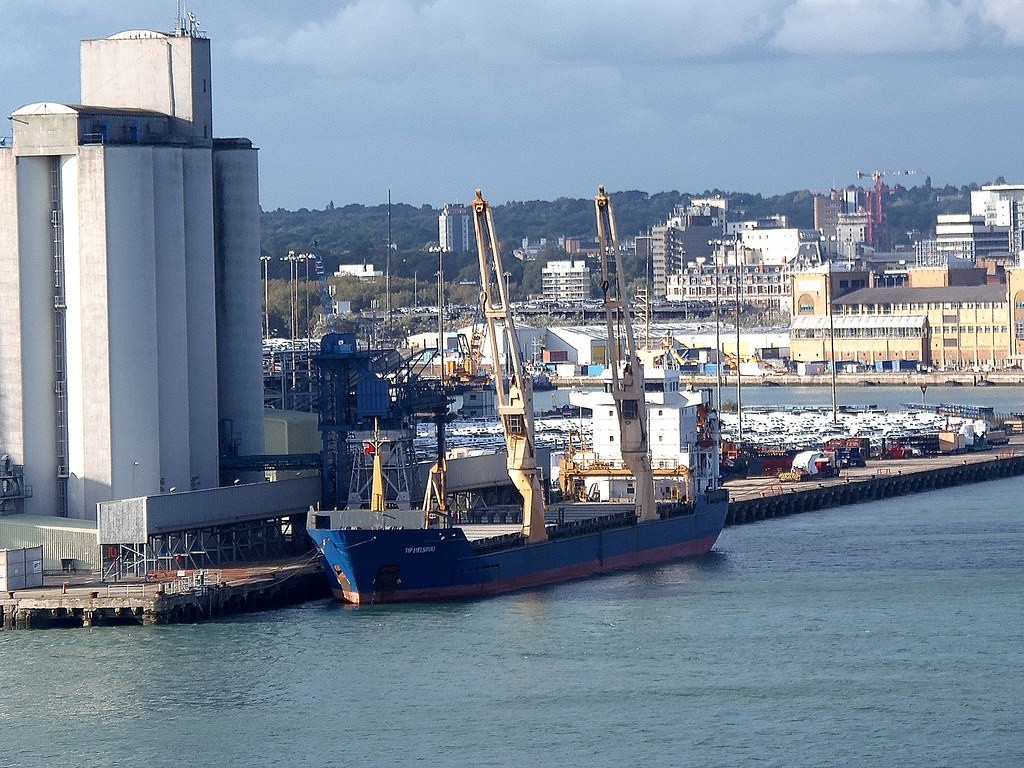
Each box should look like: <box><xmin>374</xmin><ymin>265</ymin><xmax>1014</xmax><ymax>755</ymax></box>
<box><xmin>304</xmin><ymin>184</ymin><xmax>729</xmax><ymax>608</ymax></box>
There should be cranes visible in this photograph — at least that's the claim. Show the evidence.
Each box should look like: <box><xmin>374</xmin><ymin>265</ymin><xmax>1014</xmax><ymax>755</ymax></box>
<box><xmin>809</xmin><ymin>187</ymin><xmax>898</xmax><ymax>247</ymax></box>
<box><xmin>856</xmin><ymin>169</ymin><xmax>926</xmax><ymax>222</ymax></box>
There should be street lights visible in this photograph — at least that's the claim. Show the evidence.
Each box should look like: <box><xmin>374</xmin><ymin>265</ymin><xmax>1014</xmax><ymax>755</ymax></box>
<box><xmin>260</xmin><ymin>256</ymin><xmax>271</xmax><ymax>339</ymax></box>
<box><xmin>279</xmin><ymin>249</ymin><xmax>316</xmax><ymax>412</ymax></box>
